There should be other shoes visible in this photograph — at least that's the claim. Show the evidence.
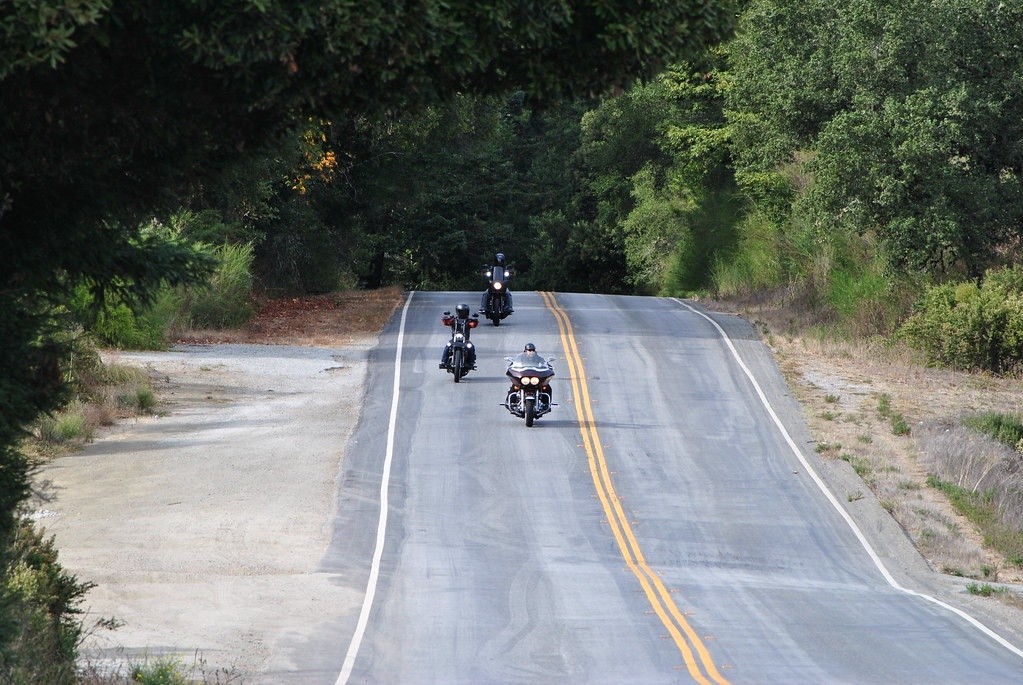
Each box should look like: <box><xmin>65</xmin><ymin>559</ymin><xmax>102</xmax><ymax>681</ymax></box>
<box><xmin>479</xmin><ymin>309</ymin><xmax>486</xmax><ymax>312</ymax></box>
<box><xmin>439</xmin><ymin>362</ymin><xmax>445</xmax><ymax>366</ymax></box>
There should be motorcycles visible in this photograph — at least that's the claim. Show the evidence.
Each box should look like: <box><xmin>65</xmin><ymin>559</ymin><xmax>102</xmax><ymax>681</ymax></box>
<box><xmin>439</xmin><ymin>311</ymin><xmax>479</xmax><ymax>383</ymax></box>
<box><xmin>481</xmin><ymin>264</ymin><xmax>517</xmax><ymax>326</ymax></box>
<box><xmin>500</xmin><ymin>353</ymin><xmax>558</xmax><ymax>428</ymax></box>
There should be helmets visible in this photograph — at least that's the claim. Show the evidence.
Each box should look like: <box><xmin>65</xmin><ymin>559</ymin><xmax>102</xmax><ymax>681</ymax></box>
<box><xmin>455</xmin><ymin>303</ymin><xmax>470</xmax><ymax>317</ymax></box>
<box><xmin>495</xmin><ymin>252</ymin><xmax>505</xmax><ymax>264</ymax></box>
<box><xmin>525</xmin><ymin>343</ymin><xmax>536</xmax><ymax>351</ymax></box>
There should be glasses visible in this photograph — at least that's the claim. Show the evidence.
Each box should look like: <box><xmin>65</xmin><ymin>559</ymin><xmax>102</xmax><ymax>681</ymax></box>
<box><xmin>528</xmin><ymin>349</ymin><xmax>534</xmax><ymax>351</ymax></box>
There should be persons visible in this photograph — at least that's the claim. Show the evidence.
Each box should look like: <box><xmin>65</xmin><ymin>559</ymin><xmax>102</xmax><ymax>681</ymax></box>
<box><xmin>506</xmin><ymin>343</ymin><xmax>552</xmax><ymax>412</ymax></box>
<box><xmin>478</xmin><ymin>253</ymin><xmax>515</xmax><ymax>314</ymax></box>
<box><xmin>438</xmin><ymin>304</ymin><xmax>478</xmax><ymax>370</ymax></box>
